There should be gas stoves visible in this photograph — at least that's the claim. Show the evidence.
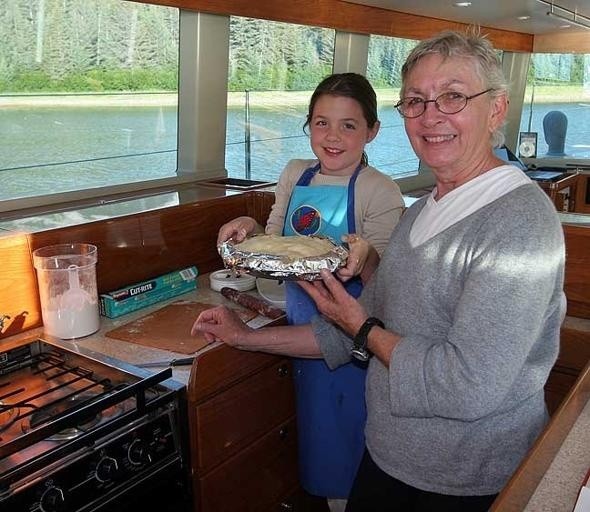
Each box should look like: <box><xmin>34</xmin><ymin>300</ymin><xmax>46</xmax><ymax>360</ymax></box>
<box><xmin>1</xmin><ymin>334</ymin><xmax>189</xmax><ymax>512</ymax></box>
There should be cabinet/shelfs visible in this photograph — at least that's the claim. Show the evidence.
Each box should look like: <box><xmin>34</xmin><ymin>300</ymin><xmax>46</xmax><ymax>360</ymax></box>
<box><xmin>190</xmin><ymin>312</ymin><xmax>301</xmax><ymax>512</ymax></box>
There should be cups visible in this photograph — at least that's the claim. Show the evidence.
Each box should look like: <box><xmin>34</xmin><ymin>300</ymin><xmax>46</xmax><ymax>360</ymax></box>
<box><xmin>32</xmin><ymin>243</ymin><xmax>101</xmax><ymax>343</ymax></box>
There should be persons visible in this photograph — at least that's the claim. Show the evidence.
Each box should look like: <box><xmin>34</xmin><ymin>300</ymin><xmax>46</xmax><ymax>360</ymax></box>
<box><xmin>216</xmin><ymin>73</ymin><xmax>406</xmax><ymax>511</ymax></box>
<box><xmin>189</xmin><ymin>24</ymin><xmax>568</xmax><ymax>511</ymax></box>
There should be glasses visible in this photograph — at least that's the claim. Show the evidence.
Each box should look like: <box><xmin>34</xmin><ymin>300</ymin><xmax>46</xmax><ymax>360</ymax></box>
<box><xmin>394</xmin><ymin>87</ymin><xmax>494</xmax><ymax>119</ymax></box>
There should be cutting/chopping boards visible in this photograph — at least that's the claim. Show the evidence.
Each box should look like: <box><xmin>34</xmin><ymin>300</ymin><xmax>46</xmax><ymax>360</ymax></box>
<box><xmin>102</xmin><ymin>297</ymin><xmax>258</xmax><ymax>357</ymax></box>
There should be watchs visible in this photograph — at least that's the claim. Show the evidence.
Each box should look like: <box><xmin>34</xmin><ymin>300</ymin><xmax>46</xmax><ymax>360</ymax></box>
<box><xmin>351</xmin><ymin>316</ymin><xmax>387</xmax><ymax>363</ymax></box>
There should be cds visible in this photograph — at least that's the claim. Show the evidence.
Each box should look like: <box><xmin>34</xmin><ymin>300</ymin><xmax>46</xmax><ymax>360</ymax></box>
<box><xmin>519</xmin><ymin>141</ymin><xmax>535</xmax><ymax>157</ymax></box>
